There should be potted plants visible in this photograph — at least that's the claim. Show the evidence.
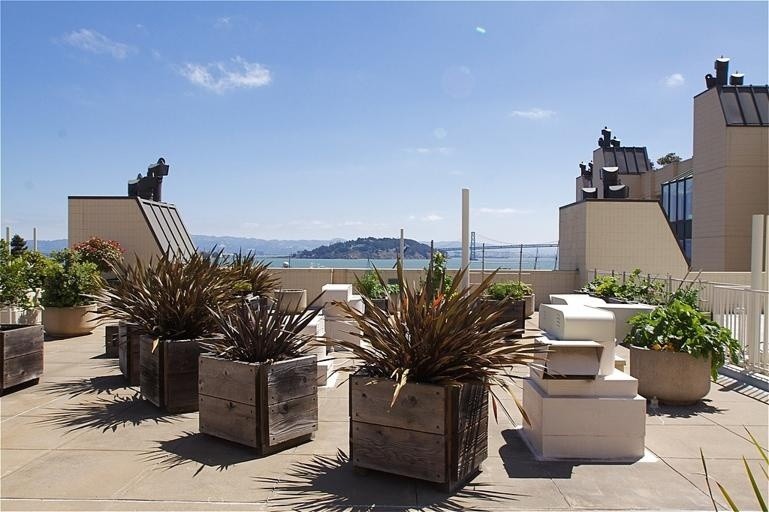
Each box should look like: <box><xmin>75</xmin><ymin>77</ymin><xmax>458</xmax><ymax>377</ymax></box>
<box><xmin>332</xmin><ymin>256</ymin><xmax>552</xmax><ymax>491</ymax></box>
<box><xmin>1</xmin><ymin>235</ymin><xmax>123</xmax><ymax>392</ymax></box>
<box><xmin>102</xmin><ymin>248</ymin><xmax>323</xmax><ymax>447</ymax></box>
<box><xmin>356</xmin><ymin>269</ymin><xmax>536</xmax><ymax>340</ymax></box>
<box><xmin>623</xmin><ymin>295</ymin><xmax>742</xmax><ymax>401</ymax></box>
